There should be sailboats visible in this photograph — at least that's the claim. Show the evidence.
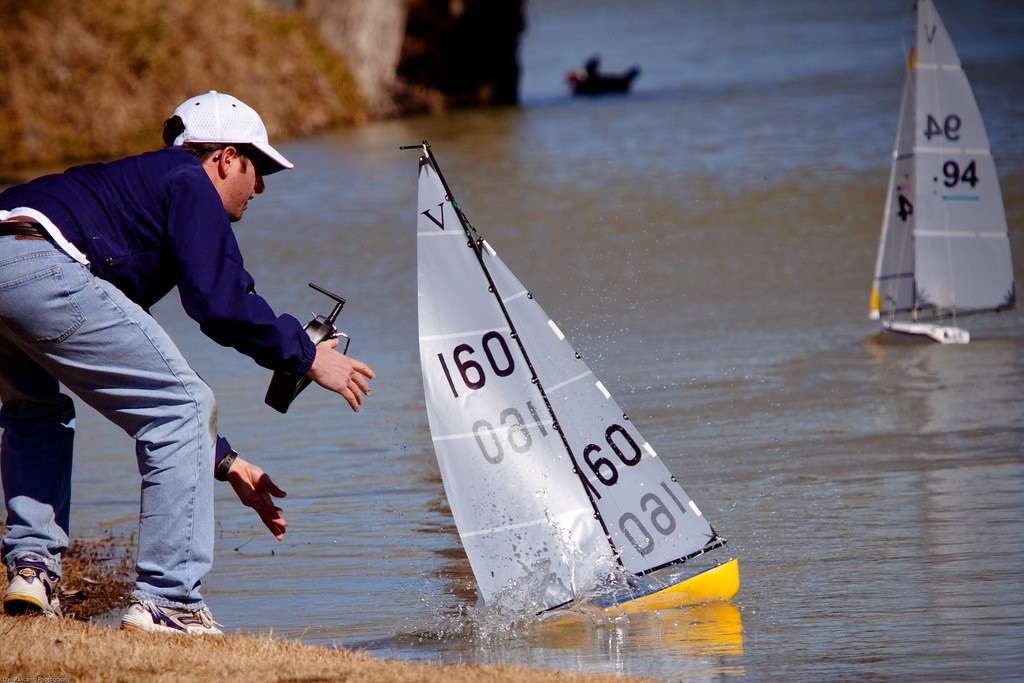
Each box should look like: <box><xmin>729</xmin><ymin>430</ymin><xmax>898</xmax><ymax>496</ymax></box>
<box><xmin>867</xmin><ymin>0</ymin><xmax>1018</xmax><ymax>346</ymax></box>
<box><xmin>393</xmin><ymin>139</ymin><xmax>740</xmax><ymax>624</ymax></box>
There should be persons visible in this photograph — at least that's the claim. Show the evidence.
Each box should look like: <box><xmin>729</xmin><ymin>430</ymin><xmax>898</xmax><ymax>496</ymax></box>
<box><xmin>0</xmin><ymin>90</ymin><xmax>375</xmax><ymax>634</ymax></box>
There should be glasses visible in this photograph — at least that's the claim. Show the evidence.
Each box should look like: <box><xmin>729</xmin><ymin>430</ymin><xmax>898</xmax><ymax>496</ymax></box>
<box><xmin>215</xmin><ymin>149</ymin><xmax>266</xmax><ymax>194</ymax></box>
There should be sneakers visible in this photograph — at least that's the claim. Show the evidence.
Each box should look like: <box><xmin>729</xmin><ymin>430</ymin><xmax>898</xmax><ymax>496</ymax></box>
<box><xmin>3</xmin><ymin>557</ymin><xmax>64</xmax><ymax>619</ymax></box>
<box><xmin>121</xmin><ymin>596</ymin><xmax>224</xmax><ymax>636</ymax></box>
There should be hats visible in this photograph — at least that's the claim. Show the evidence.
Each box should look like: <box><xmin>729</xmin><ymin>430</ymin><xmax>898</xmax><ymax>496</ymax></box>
<box><xmin>167</xmin><ymin>90</ymin><xmax>294</xmax><ymax>175</ymax></box>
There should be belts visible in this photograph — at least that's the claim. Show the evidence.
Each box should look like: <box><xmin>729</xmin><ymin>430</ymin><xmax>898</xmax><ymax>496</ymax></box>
<box><xmin>0</xmin><ymin>221</ymin><xmax>46</xmax><ymax>237</ymax></box>
